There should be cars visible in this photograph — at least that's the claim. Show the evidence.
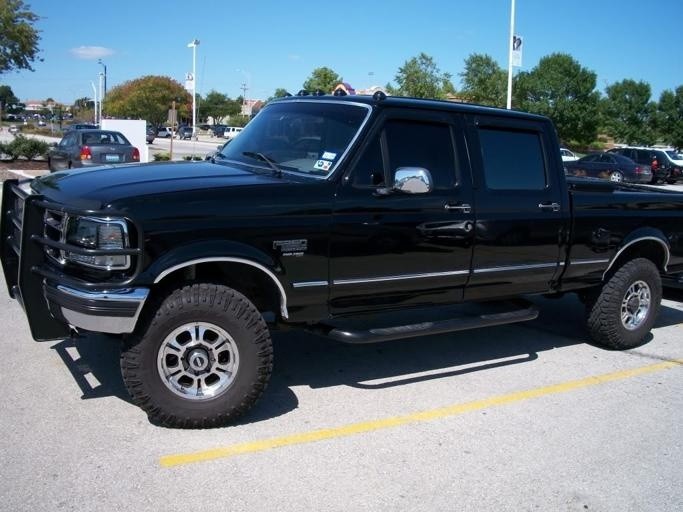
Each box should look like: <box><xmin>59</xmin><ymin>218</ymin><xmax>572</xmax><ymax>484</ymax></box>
<box><xmin>63</xmin><ymin>118</ymin><xmax>247</xmax><ymax>148</ymax></box>
<box><xmin>557</xmin><ymin>143</ymin><xmax>683</xmax><ymax>193</ymax></box>
<box><xmin>44</xmin><ymin>128</ymin><xmax>140</xmax><ymax>176</ymax></box>
<box><xmin>2</xmin><ymin>112</ymin><xmax>73</xmax><ymax>136</ymax></box>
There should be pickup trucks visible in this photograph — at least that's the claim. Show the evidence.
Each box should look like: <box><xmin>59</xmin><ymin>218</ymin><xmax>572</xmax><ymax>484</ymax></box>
<box><xmin>2</xmin><ymin>88</ymin><xmax>682</xmax><ymax>426</ymax></box>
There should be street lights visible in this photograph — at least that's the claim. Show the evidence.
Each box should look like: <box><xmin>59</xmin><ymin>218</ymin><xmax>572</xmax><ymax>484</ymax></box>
<box><xmin>187</xmin><ymin>37</ymin><xmax>199</xmax><ymax>140</ymax></box>
<box><xmin>95</xmin><ymin>72</ymin><xmax>103</xmax><ymax>126</ymax></box>
